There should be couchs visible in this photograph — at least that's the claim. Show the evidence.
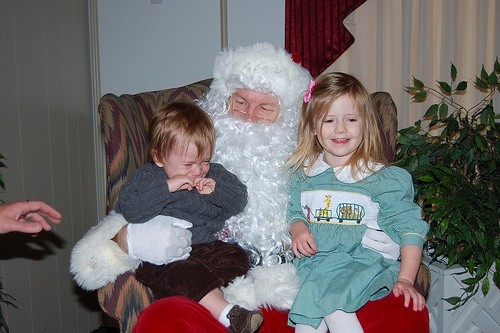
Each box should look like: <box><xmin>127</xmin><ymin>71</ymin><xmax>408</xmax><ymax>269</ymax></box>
<box><xmin>97</xmin><ymin>74</ymin><xmax>432</xmax><ymax>333</ymax></box>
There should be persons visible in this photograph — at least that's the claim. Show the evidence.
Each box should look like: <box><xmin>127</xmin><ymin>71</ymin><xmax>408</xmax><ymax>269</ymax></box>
<box><xmin>114</xmin><ymin>98</ymin><xmax>263</xmax><ymax>333</ymax></box>
<box><xmin>0</xmin><ymin>201</ymin><xmax>62</xmax><ymax>237</ymax></box>
<box><xmin>70</xmin><ymin>42</ymin><xmax>431</xmax><ymax>333</ymax></box>
<box><xmin>274</xmin><ymin>72</ymin><xmax>430</xmax><ymax>333</ymax></box>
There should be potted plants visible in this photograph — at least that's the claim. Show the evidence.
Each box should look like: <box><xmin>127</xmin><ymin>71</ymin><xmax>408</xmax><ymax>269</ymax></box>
<box><xmin>388</xmin><ymin>56</ymin><xmax>500</xmax><ymax>333</ymax></box>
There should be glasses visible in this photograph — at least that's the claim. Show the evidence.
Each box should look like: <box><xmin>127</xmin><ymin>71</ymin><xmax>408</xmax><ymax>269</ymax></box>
<box><xmin>231</xmin><ymin>96</ymin><xmax>280</xmax><ymax>125</ymax></box>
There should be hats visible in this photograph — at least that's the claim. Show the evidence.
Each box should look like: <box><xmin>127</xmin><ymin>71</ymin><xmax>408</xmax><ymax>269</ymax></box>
<box><xmin>213</xmin><ymin>41</ymin><xmax>313</xmax><ymax>109</ymax></box>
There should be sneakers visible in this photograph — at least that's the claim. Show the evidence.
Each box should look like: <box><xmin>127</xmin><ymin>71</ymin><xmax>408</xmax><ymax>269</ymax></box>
<box><xmin>226</xmin><ymin>305</ymin><xmax>263</xmax><ymax>333</ymax></box>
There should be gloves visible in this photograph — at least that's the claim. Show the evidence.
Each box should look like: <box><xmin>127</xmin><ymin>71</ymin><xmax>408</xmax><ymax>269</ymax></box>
<box><xmin>362</xmin><ymin>228</ymin><xmax>400</xmax><ymax>262</ymax></box>
<box><xmin>127</xmin><ymin>215</ymin><xmax>193</xmax><ymax>265</ymax></box>
